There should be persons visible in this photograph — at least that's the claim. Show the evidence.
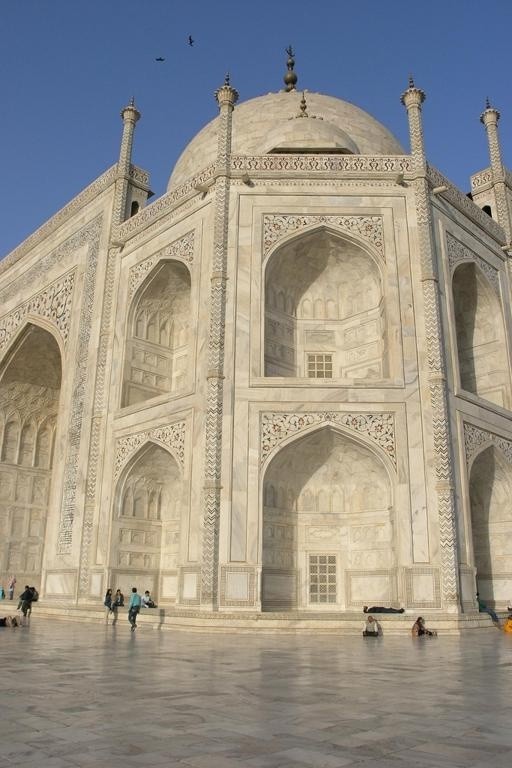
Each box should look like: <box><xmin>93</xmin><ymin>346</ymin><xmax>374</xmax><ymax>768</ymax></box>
<box><xmin>477</xmin><ymin>592</ymin><xmax>498</xmax><ymax>621</ymax></box>
<box><xmin>411</xmin><ymin>616</ymin><xmax>437</xmax><ymax>638</ymax></box>
<box><xmin>0</xmin><ymin>615</ymin><xmax>23</xmax><ymax>632</ymax></box>
<box><xmin>22</xmin><ymin>587</ymin><xmax>39</xmax><ymax>616</ymax></box>
<box><xmin>7</xmin><ymin>574</ymin><xmax>16</xmax><ymax>600</ymax></box>
<box><xmin>17</xmin><ymin>585</ymin><xmax>30</xmax><ymax>610</ymax></box>
<box><xmin>362</xmin><ymin>616</ymin><xmax>379</xmax><ymax>637</ymax></box>
<box><xmin>104</xmin><ymin>587</ymin><xmax>155</xmax><ymax>631</ymax></box>
<box><xmin>363</xmin><ymin>605</ymin><xmax>405</xmax><ymax>613</ymax></box>
